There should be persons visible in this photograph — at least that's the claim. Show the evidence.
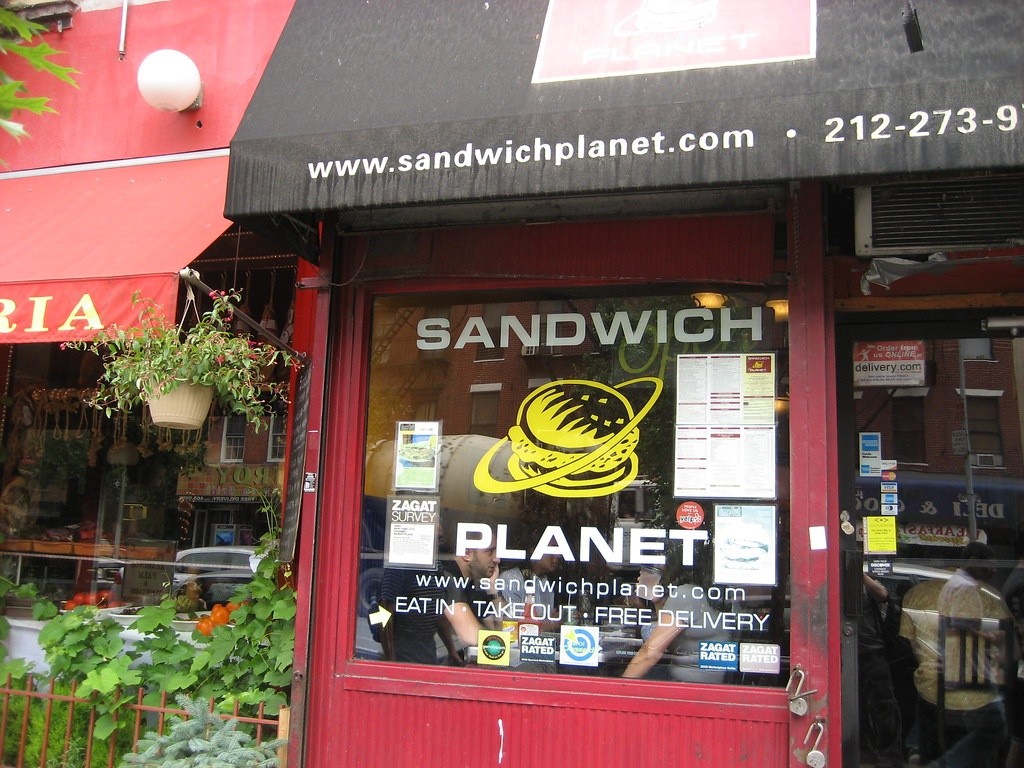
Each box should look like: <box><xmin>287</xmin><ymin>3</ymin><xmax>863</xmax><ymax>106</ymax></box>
<box><xmin>857</xmin><ymin>541</ymin><xmax>1024</xmax><ymax>768</ymax></box>
<box><xmin>439</xmin><ymin>527</ymin><xmax>519</xmax><ymax>647</ymax></box>
<box><xmin>378</xmin><ymin>517</ymin><xmax>463</xmax><ymax>667</ymax></box>
<box><xmin>497</xmin><ymin>527</ymin><xmax>566</xmax><ymax>637</ymax></box>
<box><xmin>620</xmin><ymin>546</ymin><xmax>731</xmax><ymax>684</ymax></box>
<box><xmin>930</xmin><ymin>542</ymin><xmax>1006</xmax><ymax>768</ymax></box>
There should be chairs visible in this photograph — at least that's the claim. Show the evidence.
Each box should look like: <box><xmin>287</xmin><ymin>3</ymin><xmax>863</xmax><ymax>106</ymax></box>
<box><xmin>918</xmin><ymin>614</ymin><xmax>1014</xmax><ymax>761</ymax></box>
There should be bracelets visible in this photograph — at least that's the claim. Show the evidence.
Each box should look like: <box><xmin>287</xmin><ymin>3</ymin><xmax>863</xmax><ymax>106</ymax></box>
<box><xmin>487</xmin><ymin>591</ymin><xmax>502</xmax><ymax>600</ymax></box>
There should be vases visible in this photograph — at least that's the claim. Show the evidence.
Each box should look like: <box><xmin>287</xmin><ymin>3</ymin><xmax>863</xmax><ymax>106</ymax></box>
<box><xmin>147</xmin><ymin>378</ymin><xmax>214</xmax><ymax>430</ymax></box>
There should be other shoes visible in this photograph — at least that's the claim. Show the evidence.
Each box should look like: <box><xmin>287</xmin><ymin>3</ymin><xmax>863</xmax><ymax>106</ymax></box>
<box><xmin>906</xmin><ymin>746</ymin><xmax>921</xmax><ymax>768</ymax></box>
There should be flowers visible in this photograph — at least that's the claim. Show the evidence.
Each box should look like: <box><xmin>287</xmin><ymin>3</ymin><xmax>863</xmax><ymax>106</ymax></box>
<box><xmin>60</xmin><ymin>287</ymin><xmax>305</xmax><ymax>435</ymax></box>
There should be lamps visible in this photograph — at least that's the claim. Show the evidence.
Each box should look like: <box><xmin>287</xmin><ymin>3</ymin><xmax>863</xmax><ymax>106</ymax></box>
<box><xmin>137</xmin><ymin>49</ymin><xmax>203</xmax><ymax>114</ymax></box>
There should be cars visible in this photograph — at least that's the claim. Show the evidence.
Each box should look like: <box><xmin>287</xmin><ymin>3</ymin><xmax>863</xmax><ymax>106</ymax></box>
<box><xmin>95</xmin><ymin>542</ymin><xmax>275</xmax><ymax>623</ymax></box>
<box><xmin>863</xmin><ymin>559</ymin><xmax>1019</xmax><ymax>714</ymax></box>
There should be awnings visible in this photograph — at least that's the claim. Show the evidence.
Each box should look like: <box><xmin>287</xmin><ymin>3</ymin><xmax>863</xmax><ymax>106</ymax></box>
<box><xmin>0</xmin><ymin>149</ymin><xmax>233</xmax><ymax>345</ymax></box>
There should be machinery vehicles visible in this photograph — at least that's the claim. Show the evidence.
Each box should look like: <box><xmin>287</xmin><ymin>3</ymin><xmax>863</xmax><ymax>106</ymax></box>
<box><xmin>358</xmin><ymin>432</ymin><xmax>662</xmax><ymax>625</ymax></box>
